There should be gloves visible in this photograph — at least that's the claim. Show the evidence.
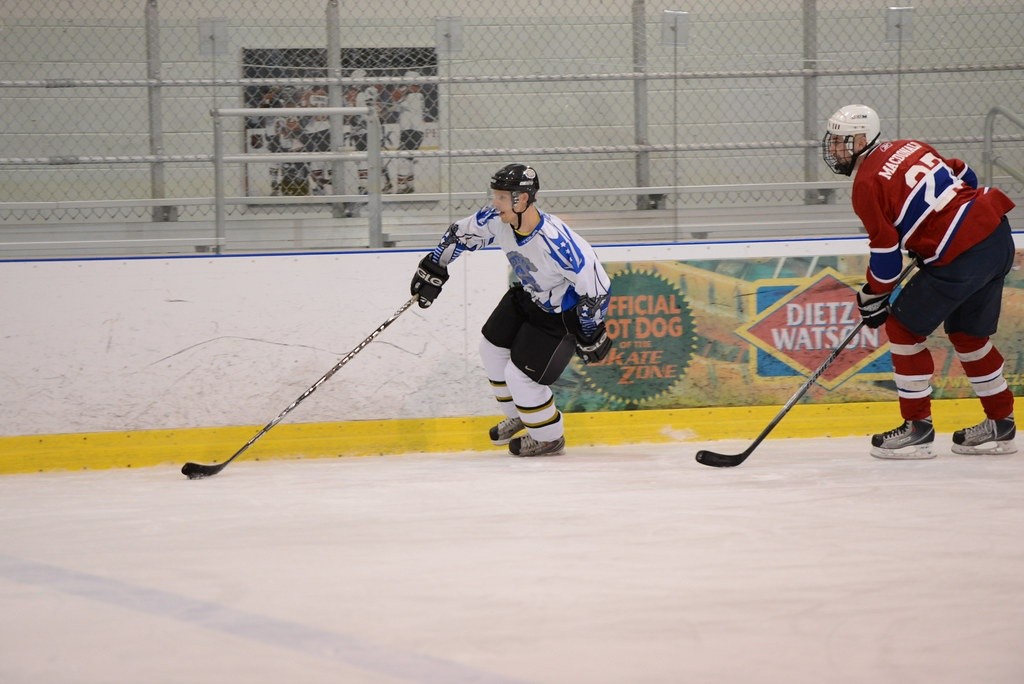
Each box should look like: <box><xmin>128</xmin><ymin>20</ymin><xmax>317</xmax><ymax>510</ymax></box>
<box><xmin>411</xmin><ymin>253</ymin><xmax>449</xmax><ymax>308</ymax></box>
<box><xmin>574</xmin><ymin>321</ymin><xmax>612</xmax><ymax>364</ymax></box>
<box><xmin>855</xmin><ymin>282</ymin><xmax>890</xmax><ymax>329</ymax></box>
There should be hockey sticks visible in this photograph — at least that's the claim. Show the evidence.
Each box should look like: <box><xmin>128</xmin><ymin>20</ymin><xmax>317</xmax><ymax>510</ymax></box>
<box><xmin>694</xmin><ymin>252</ymin><xmax>919</xmax><ymax>471</ymax></box>
<box><xmin>181</xmin><ymin>291</ymin><xmax>423</xmax><ymax>480</ymax></box>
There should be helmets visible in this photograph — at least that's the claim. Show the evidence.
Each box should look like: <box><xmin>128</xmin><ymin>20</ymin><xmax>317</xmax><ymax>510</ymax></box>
<box><xmin>349</xmin><ymin>70</ymin><xmax>366</xmax><ymax>79</ymax></box>
<box><xmin>491</xmin><ymin>162</ymin><xmax>540</xmax><ymax>203</ymax></box>
<box><xmin>404</xmin><ymin>70</ymin><xmax>422</xmax><ymax>80</ymax></box>
<box><xmin>822</xmin><ymin>105</ymin><xmax>882</xmax><ymax>175</ymax></box>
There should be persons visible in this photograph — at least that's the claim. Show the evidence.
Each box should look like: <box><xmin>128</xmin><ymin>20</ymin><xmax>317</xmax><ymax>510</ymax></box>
<box><xmin>261</xmin><ymin>67</ymin><xmax>424</xmax><ymax>197</ymax></box>
<box><xmin>410</xmin><ymin>164</ymin><xmax>612</xmax><ymax>456</ymax></box>
<box><xmin>822</xmin><ymin>105</ymin><xmax>1017</xmax><ymax>459</ymax></box>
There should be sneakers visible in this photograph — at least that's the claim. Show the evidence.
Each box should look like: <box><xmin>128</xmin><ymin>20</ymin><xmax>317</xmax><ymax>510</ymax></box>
<box><xmin>489</xmin><ymin>415</ymin><xmax>526</xmax><ymax>446</ymax></box>
<box><xmin>870</xmin><ymin>416</ymin><xmax>935</xmax><ymax>460</ymax></box>
<box><xmin>951</xmin><ymin>410</ymin><xmax>1019</xmax><ymax>455</ymax></box>
<box><xmin>508</xmin><ymin>432</ymin><xmax>567</xmax><ymax>458</ymax></box>
<box><xmin>312</xmin><ymin>184</ymin><xmax>326</xmax><ymax>195</ymax></box>
<box><xmin>357</xmin><ymin>185</ymin><xmax>370</xmax><ymax>195</ymax></box>
<box><xmin>396</xmin><ymin>182</ymin><xmax>414</xmax><ymax>194</ymax></box>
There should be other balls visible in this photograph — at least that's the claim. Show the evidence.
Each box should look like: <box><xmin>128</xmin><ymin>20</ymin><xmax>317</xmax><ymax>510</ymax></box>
<box><xmin>186</xmin><ymin>472</ymin><xmax>205</xmax><ymax>480</ymax></box>
